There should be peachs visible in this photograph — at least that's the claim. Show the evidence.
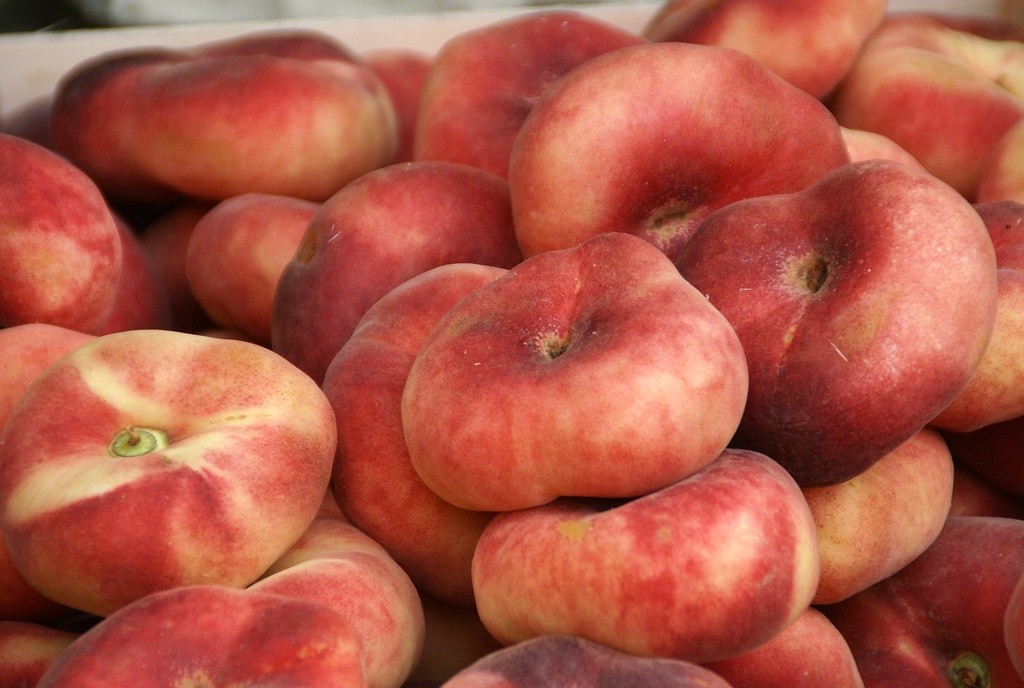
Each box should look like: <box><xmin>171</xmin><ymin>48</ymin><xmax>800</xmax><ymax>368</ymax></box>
<box><xmin>0</xmin><ymin>0</ymin><xmax>1024</xmax><ymax>688</ymax></box>
